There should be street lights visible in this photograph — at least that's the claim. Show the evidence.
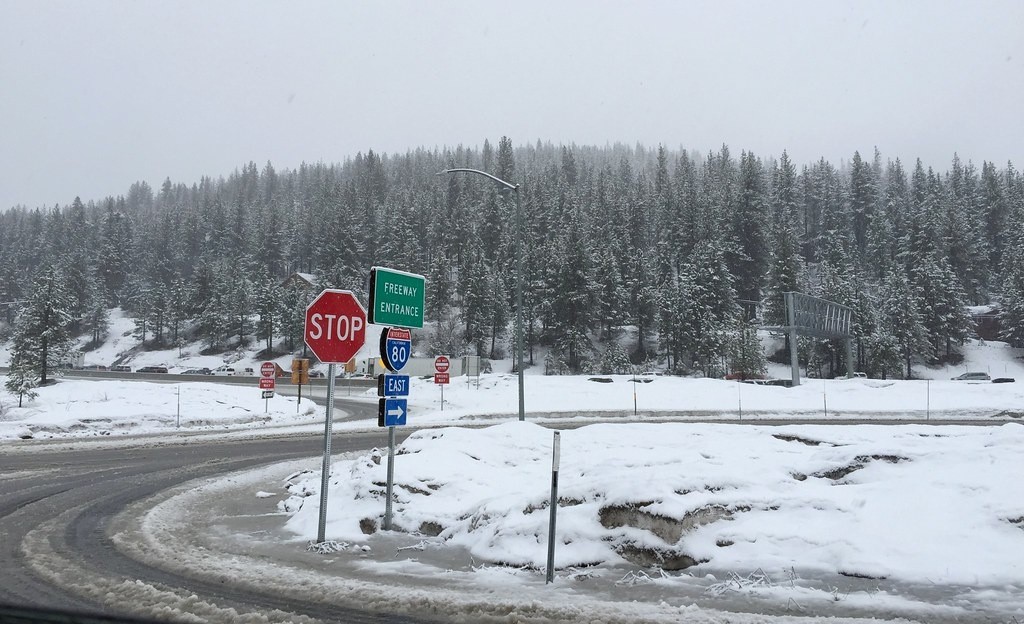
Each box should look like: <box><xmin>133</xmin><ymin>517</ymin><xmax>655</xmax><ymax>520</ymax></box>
<box><xmin>435</xmin><ymin>168</ymin><xmax>525</xmax><ymax>423</ymax></box>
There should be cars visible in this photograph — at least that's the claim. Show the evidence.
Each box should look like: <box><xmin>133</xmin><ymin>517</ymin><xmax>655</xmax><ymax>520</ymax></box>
<box><xmin>308</xmin><ymin>369</ymin><xmax>326</xmax><ymax>378</ymax></box>
<box><xmin>348</xmin><ymin>373</ymin><xmax>374</xmax><ymax>379</ymax></box>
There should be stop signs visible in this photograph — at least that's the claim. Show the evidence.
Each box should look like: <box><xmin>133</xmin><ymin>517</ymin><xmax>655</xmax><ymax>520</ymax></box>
<box><xmin>435</xmin><ymin>356</ymin><xmax>450</xmax><ymax>372</ymax></box>
<box><xmin>259</xmin><ymin>361</ymin><xmax>276</xmax><ymax>378</ymax></box>
<box><xmin>304</xmin><ymin>288</ymin><xmax>368</xmax><ymax>365</ymax></box>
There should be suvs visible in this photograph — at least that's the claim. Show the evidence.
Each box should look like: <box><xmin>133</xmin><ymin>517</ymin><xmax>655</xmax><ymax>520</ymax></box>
<box><xmin>181</xmin><ymin>369</ymin><xmax>206</xmax><ymax>375</ymax></box>
<box><xmin>136</xmin><ymin>367</ymin><xmax>168</xmax><ymax>373</ymax></box>
<box><xmin>845</xmin><ymin>372</ymin><xmax>868</xmax><ymax>380</ymax></box>
<box><xmin>743</xmin><ymin>378</ymin><xmax>791</xmax><ymax>388</ymax></box>
<box><xmin>87</xmin><ymin>365</ymin><xmax>106</xmax><ymax>371</ymax></box>
<box><xmin>111</xmin><ymin>365</ymin><xmax>131</xmax><ymax>372</ymax></box>
<box><xmin>951</xmin><ymin>371</ymin><xmax>991</xmax><ymax>380</ymax></box>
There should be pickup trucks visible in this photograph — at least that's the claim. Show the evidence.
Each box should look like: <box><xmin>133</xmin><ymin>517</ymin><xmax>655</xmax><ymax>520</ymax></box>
<box><xmin>211</xmin><ymin>367</ymin><xmax>239</xmax><ymax>376</ymax></box>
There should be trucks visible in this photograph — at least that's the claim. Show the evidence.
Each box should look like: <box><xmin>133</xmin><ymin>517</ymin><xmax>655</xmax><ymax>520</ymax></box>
<box><xmin>41</xmin><ymin>351</ymin><xmax>85</xmax><ymax>371</ymax></box>
<box><xmin>356</xmin><ymin>356</ymin><xmax>465</xmax><ymax>378</ymax></box>
